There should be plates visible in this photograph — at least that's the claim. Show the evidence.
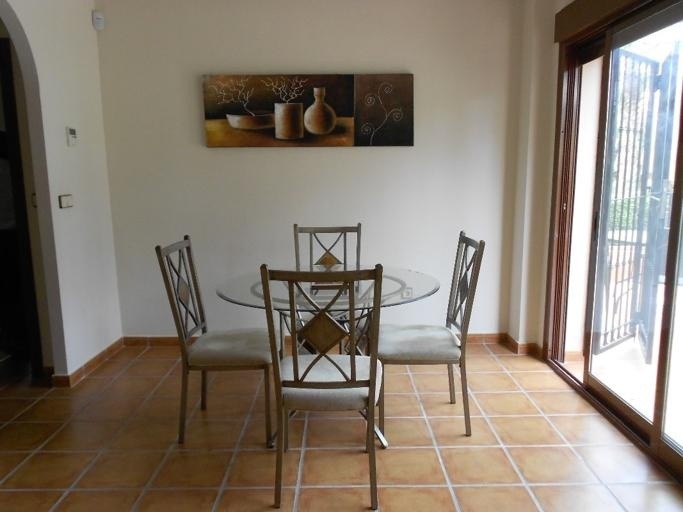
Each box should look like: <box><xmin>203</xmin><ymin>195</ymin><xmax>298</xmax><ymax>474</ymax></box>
<box><xmin>228</xmin><ymin>111</ymin><xmax>274</xmax><ymax>129</ymax></box>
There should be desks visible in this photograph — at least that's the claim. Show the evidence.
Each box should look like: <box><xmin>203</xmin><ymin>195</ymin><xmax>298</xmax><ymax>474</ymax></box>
<box><xmin>216</xmin><ymin>264</ymin><xmax>440</xmax><ymax>450</ymax></box>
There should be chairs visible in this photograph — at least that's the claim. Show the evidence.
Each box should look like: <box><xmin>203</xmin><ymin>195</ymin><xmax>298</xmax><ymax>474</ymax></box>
<box><xmin>378</xmin><ymin>231</ymin><xmax>486</xmax><ymax>439</ymax></box>
<box><xmin>293</xmin><ymin>223</ymin><xmax>362</xmax><ymax>355</ymax></box>
<box><xmin>258</xmin><ymin>263</ymin><xmax>383</xmax><ymax>510</ymax></box>
<box><xmin>154</xmin><ymin>235</ymin><xmax>288</xmax><ymax>448</ymax></box>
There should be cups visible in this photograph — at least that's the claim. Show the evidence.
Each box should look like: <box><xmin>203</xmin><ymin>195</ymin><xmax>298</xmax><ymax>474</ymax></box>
<box><xmin>274</xmin><ymin>103</ymin><xmax>305</xmax><ymax>140</ymax></box>
